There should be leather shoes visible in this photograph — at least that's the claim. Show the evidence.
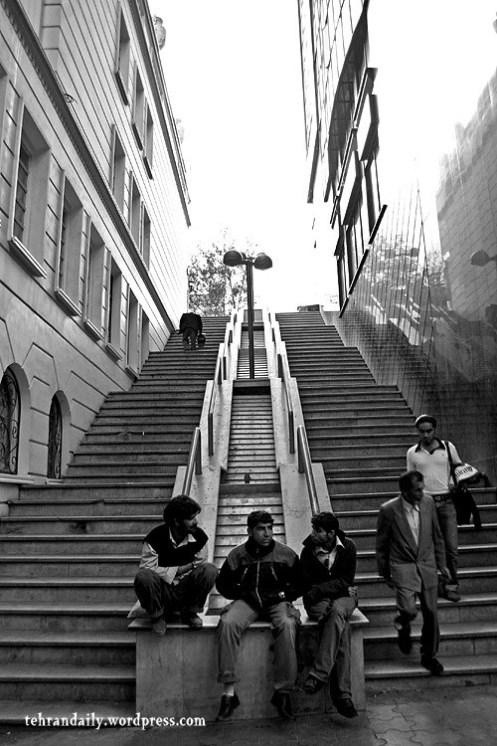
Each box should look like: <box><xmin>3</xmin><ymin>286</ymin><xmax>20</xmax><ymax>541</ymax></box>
<box><xmin>271</xmin><ymin>694</ymin><xmax>296</xmax><ymax>723</ymax></box>
<box><xmin>333</xmin><ymin>697</ymin><xmax>360</xmax><ymax>719</ymax></box>
<box><xmin>217</xmin><ymin>691</ymin><xmax>240</xmax><ymax>721</ymax></box>
<box><xmin>301</xmin><ymin>676</ymin><xmax>324</xmax><ymax>694</ymax></box>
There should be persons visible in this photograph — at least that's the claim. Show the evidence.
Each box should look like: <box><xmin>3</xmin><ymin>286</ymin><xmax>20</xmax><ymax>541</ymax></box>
<box><xmin>405</xmin><ymin>413</ymin><xmax>465</xmax><ymax>602</ymax></box>
<box><xmin>298</xmin><ymin>511</ymin><xmax>358</xmax><ymax>719</ymax></box>
<box><xmin>178</xmin><ymin>312</ymin><xmax>202</xmax><ymax>351</ymax></box>
<box><xmin>214</xmin><ymin>511</ymin><xmax>310</xmax><ymax>723</ymax></box>
<box><xmin>135</xmin><ymin>494</ymin><xmax>218</xmax><ymax>636</ymax></box>
<box><xmin>375</xmin><ymin>470</ymin><xmax>451</xmax><ymax>675</ymax></box>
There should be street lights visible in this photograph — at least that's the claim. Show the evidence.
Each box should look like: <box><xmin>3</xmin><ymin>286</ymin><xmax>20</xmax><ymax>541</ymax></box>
<box><xmin>223</xmin><ymin>250</ymin><xmax>273</xmax><ymax>378</ymax></box>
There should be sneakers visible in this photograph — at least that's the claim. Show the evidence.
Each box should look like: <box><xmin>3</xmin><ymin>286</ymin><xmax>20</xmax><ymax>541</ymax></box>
<box><xmin>420</xmin><ymin>656</ymin><xmax>444</xmax><ymax>675</ymax></box>
<box><xmin>399</xmin><ymin>633</ymin><xmax>412</xmax><ymax>655</ymax></box>
<box><xmin>152</xmin><ymin>616</ymin><xmax>166</xmax><ymax>636</ymax></box>
<box><xmin>182</xmin><ymin>613</ymin><xmax>202</xmax><ymax>628</ymax></box>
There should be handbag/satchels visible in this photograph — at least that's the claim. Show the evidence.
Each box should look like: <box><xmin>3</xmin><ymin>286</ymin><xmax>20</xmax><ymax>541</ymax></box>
<box><xmin>453</xmin><ymin>485</ymin><xmax>480</xmax><ymax>525</ymax></box>
